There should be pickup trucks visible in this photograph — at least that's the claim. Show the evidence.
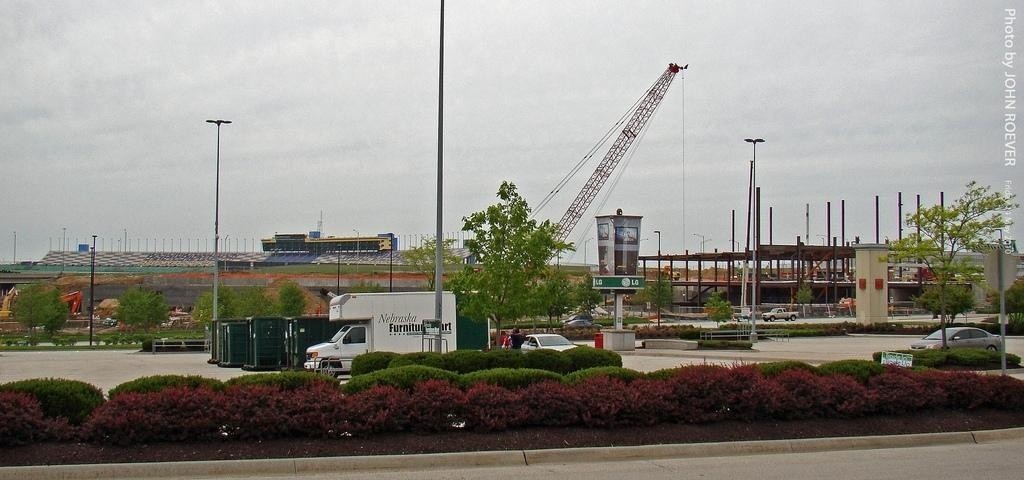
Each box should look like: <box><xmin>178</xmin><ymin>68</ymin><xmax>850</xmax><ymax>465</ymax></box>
<box><xmin>760</xmin><ymin>305</ymin><xmax>801</xmax><ymax>322</ymax></box>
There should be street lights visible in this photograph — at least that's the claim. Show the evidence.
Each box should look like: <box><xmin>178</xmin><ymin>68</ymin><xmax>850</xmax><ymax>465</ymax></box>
<box><xmin>5</xmin><ymin>227</ymin><xmax>266</xmax><ymax>273</ymax></box>
<box><xmin>582</xmin><ymin>230</ymin><xmax>744</xmax><ymax>278</ymax></box>
<box><xmin>343</xmin><ymin>224</ymin><xmax>477</xmax><ymax>277</ymax></box>
<box><xmin>744</xmin><ymin>137</ymin><xmax>765</xmax><ymax>342</ymax></box>
<box><xmin>205</xmin><ymin>117</ymin><xmax>234</xmax><ymax>320</ymax></box>
<box><xmin>651</xmin><ymin>230</ymin><xmax>662</xmax><ymax>326</ymax></box>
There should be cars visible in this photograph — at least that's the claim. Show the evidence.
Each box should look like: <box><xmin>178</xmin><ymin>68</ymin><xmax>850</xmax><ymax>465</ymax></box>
<box><xmin>908</xmin><ymin>323</ymin><xmax>1007</xmax><ymax>357</ymax></box>
<box><xmin>561</xmin><ymin>310</ymin><xmax>603</xmax><ymax>330</ymax></box>
<box><xmin>504</xmin><ymin>334</ymin><xmax>576</xmax><ymax>355</ymax></box>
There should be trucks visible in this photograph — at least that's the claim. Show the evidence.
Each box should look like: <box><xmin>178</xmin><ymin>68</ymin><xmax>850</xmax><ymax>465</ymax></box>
<box><xmin>647</xmin><ymin>265</ymin><xmax>680</xmax><ymax>281</ymax></box>
<box><xmin>820</xmin><ymin>296</ymin><xmax>895</xmax><ymax>316</ymax></box>
<box><xmin>300</xmin><ymin>291</ymin><xmax>457</xmax><ymax>381</ymax></box>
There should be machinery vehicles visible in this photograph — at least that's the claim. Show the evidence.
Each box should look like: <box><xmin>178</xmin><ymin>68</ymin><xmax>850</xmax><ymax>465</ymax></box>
<box><xmin>0</xmin><ymin>285</ymin><xmax>201</xmax><ymax>343</ymax></box>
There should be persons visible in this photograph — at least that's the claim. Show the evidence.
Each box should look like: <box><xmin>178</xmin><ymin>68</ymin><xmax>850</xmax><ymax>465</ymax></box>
<box><xmin>504</xmin><ymin>333</ymin><xmax>513</xmax><ymax>349</ymax></box>
<box><xmin>511</xmin><ymin>327</ymin><xmax>525</xmax><ymax>349</ymax></box>
<box><xmin>499</xmin><ymin>331</ymin><xmax>510</xmax><ymax>347</ymax></box>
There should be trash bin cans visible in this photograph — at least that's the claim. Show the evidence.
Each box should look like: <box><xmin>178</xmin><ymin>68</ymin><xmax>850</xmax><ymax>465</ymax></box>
<box><xmin>595</xmin><ymin>333</ymin><xmax>603</xmax><ymax>348</ymax></box>
